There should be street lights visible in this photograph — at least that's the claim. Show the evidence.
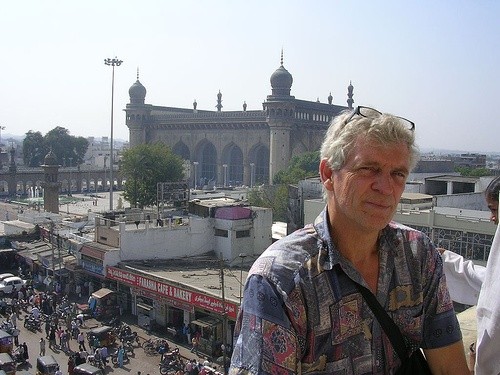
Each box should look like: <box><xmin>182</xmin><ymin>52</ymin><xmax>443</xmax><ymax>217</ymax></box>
<box><xmin>103</xmin><ymin>55</ymin><xmax>124</xmax><ymax>210</ymax></box>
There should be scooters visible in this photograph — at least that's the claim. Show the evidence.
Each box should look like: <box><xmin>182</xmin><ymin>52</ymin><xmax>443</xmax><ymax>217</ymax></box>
<box><xmin>160</xmin><ymin>348</ymin><xmax>184</xmax><ymax>374</ymax></box>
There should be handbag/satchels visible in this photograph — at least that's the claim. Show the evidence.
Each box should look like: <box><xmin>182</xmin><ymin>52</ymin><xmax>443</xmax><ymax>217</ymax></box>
<box><xmin>394</xmin><ymin>349</ymin><xmax>433</xmax><ymax>375</ymax></box>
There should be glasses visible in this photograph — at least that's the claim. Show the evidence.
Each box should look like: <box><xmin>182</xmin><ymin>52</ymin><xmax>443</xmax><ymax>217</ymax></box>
<box><xmin>343</xmin><ymin>105</ymin><xmax>416</xmax><ymax>135</ymax></box>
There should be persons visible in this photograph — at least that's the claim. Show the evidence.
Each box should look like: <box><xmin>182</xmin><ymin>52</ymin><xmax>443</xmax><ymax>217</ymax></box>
<box><xmin>0</xmin><ymin>266</ymin><xmax>231</xmax><ymax>375</ymax></box>
<box><xmin>436</xmin><ymin>177</ymin><xmax>500</xmax><ymax>375</ymax></box>
<box><xmin>227</xmin><ymin>108</ymin><xmax>471</xmax><ymax>375</ymax></box>
<box><xmin>5</xmin><ymin>211</ymin><xmax>8</xmax><ymax>219</ymax></box>
<box><xmin>20</xmin><ymin>205</ymin><xmax>24</xmax><ymax>214</ymax></box>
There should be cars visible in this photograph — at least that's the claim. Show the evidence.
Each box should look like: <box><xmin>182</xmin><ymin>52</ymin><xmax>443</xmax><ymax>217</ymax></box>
<box><xmin>0</xmin><ymin>277</ymin><xmax>27</xmax><ymax>296</ymax></box>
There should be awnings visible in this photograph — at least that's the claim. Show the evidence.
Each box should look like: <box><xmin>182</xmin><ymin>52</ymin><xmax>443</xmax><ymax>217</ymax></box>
<box><xmin>91</xmin><ymin>287</ymin><xmax>113</xmax><ymax>299</ymax></box>
<box><xmin>191</xmin><ymin>316</ymin><xmax>222</xmax><ymax>328</ymax></box>
